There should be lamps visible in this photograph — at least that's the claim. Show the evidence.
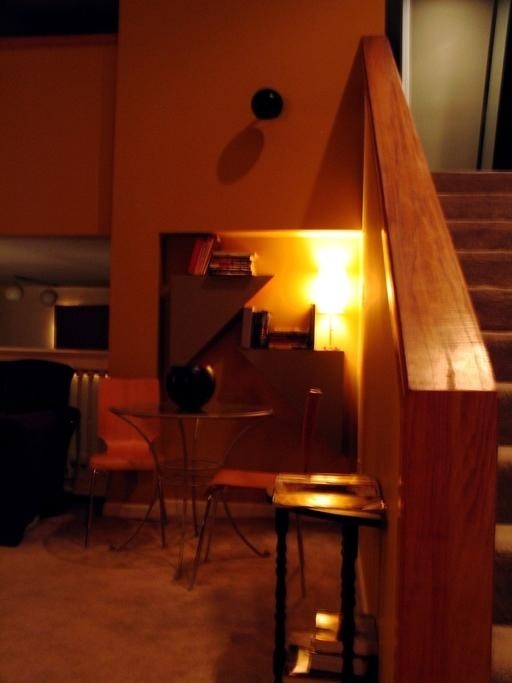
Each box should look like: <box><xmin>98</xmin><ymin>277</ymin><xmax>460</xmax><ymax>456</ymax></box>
<box><xmin>189</xmin><ymin>389</ymin><xmax>323</xmax><ymax>602</ymax></box>
<box><xmin>84</xmin><ymin>369</ymin><xmax>168</xmax><ymax>552</ymax></box>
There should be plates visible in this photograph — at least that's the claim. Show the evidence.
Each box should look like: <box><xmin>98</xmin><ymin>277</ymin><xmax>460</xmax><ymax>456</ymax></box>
<box><xmin>269</xmin><ymin>470</ymin><xmax>388</xmax><ymax>683</ymax></box>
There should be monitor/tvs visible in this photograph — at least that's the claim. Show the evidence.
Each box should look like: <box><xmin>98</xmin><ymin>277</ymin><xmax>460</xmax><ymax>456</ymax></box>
<box><xmin>186</xmin><ymin>238</ymin><xmax>311</xmax><ymax>350</ymax></box>
